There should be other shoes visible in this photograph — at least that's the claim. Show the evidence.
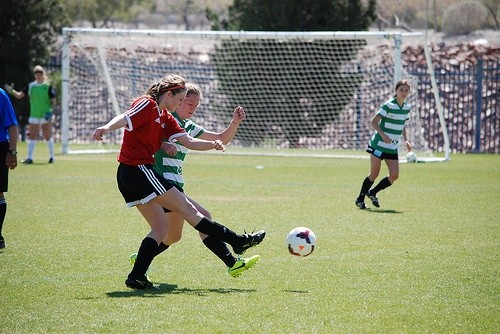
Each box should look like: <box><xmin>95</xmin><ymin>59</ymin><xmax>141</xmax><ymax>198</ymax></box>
<box><xmin>0</xmin><ymin>237</ymin><xmax>5</xmax><ymax>252</ymax></box>
<box><xmin>21</xmin><ymin>159</ymin><xmax>32</xmax><ymax>164</ymax></box>
<box><xmin>48</xmin><ymin>157</ymin><xmax>53</xmax><ymax>164</ymax></box>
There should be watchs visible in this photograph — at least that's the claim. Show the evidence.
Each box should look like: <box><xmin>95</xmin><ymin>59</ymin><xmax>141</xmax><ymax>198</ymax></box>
<box><xmin>8</xmin><ymin>149</ymin><xmax>17</xmax><ymax>155</ymax></box>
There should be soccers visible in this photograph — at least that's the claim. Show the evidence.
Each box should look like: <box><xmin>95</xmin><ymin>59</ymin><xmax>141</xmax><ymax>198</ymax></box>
<box><xmin>286</xmin><ymin>227</ymin><xmax>316</xmax><ymax>256</ymax></box>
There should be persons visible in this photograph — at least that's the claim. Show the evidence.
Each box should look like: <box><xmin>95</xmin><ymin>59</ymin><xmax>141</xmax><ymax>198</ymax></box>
<box><xmin>0</xmin><ymin>88</ymin><xmax>18</xmax><ymax>250</ymax></box>
<box><xmin>5</xmin><ymin>65</ymin><xmax>55</xmax><ymax>163</ymax></box>
<box><xmin>129</xmin><ymin>83</ymin><xmax>260</xmax><ymax>278</ymax></box>
<box><xmin>92</xmin><ymin>74</ymin><xmax>266</xmax><ymax>291</ymax></box>
<box><xmin>355</xmin><ymin>80</ymin><xmax>412</xmax><ymax>209</ymax></box>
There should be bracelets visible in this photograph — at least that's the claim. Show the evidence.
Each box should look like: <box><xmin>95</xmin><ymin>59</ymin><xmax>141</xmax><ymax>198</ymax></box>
<box><xmin>10</xmin><ymin>88</ymin><xmax>16</xmax><ymax>95</ymax></box>
<box><xmin>231</xmin><ymin>120</ymin><xmax>240</xmax><ymax>126</ymax></box>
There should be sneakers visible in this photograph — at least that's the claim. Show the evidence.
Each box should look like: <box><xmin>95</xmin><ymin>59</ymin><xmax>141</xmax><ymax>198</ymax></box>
<box><xmin>125</xmin><ymin>273</ymin><xmax>160</xmax><ymax>290</ymax></box>
<box><xmin>366</xmin><ymin>190</ymin><xmax>380</xmax><ymax>207</ymax></box>
<box><xmin>355</xmin><ymin>198</ymin><xmax>366</xmax><ymax>209</ymax></box>
<box><xmin>228</xmin><ymin>255</ymin><xmax>260</xmax><ymax>279</ymax></box>
<box><xmin>129</xmin><ymin>253</ymin><xmax>138</xmax><ymax>268</ymax></box>
<box><xmin>233</xmin><ymin>230</ymin><xmax>265</xmax><ymax>255</ymax></box>
